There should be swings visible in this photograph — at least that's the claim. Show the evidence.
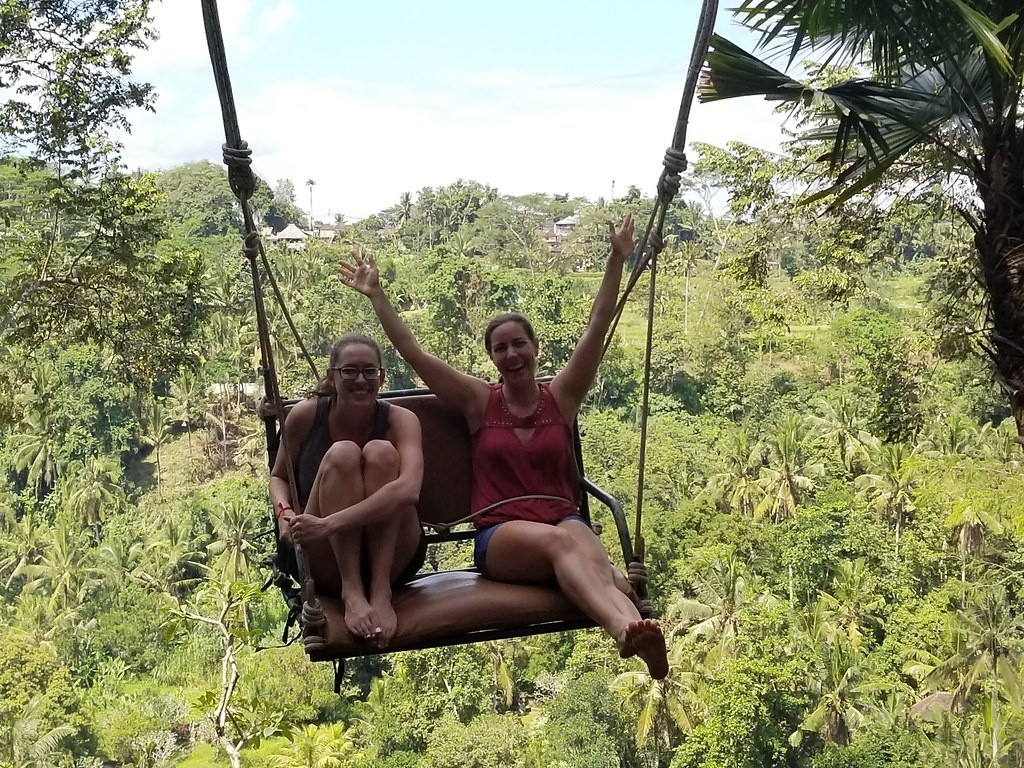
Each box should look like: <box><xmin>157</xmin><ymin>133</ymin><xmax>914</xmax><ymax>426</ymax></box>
<box><xmin>202</xmin><ymin>0</ymin><xmax>721</xmax><ymax>695</ymax></box>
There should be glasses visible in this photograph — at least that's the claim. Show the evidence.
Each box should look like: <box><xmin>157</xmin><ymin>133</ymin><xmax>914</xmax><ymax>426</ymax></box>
<box><xmin>331</xmin><ymin>367</ymin><xmax>384</xmax><ymax>380</ymax></box>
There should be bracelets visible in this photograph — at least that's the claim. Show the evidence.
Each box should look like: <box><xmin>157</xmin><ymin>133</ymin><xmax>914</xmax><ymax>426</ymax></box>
<box><xmin>276</xmin><ymin>501</ymin><xmax>293</xmax><ymax>518</ymax></box>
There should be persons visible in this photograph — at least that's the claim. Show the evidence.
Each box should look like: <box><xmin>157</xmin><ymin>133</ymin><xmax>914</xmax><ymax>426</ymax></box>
<box><xmin>269</xmin><ymin>337</ymin><xmax>427</xmax><ymax>654</ymax></box>
<box><xmin>337</xmin><ymin>213</ymin><xmax>673</xmax><ymax>681</ymax></box>
